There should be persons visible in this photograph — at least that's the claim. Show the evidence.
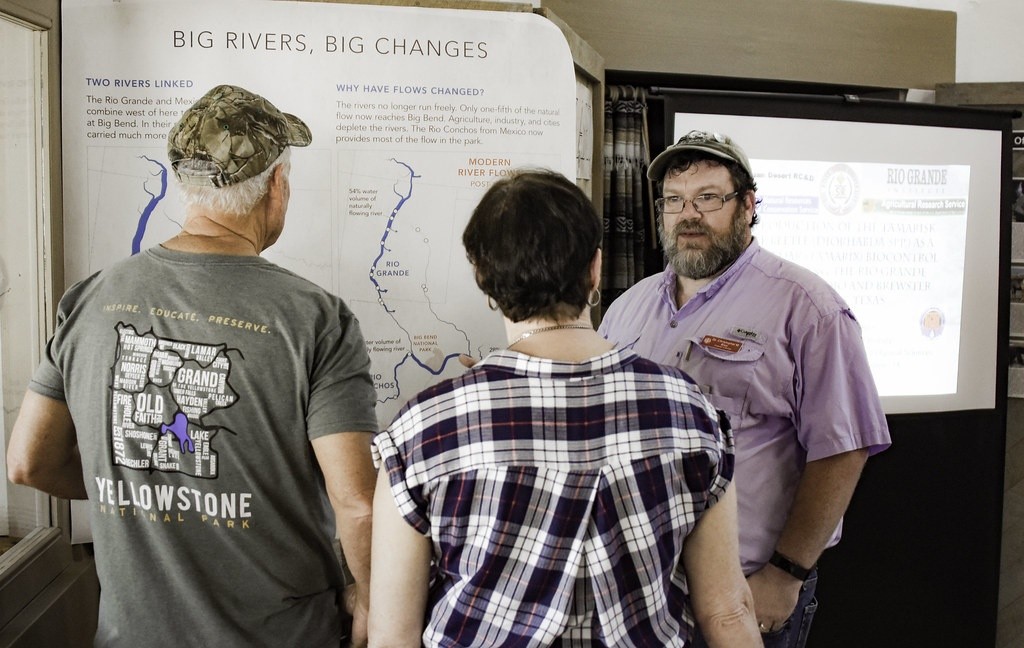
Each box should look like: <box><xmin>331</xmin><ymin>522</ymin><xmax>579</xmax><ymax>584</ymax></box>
<box><xmin>368</xmin><ymin>168</ymin><xmax>765</xmax><ymax>648</ymax></box>
<box><xmin>6</xmin><ymin>86</ymin><xmax>384</xmax><ymax>647</ymax></box>
<box><xmin>594</xmin><ymin>130</ymin><xmax>891</xmax><ymax>648</ymax></box>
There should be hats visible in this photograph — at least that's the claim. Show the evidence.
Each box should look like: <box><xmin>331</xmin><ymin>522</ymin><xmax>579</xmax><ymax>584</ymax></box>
<box><xmin>167</xmin><ymin>85</ymin><xmax>312</xmax><ymax>188</ymax></box>
<box><xmin>647</xmin><ymin>129</ymin><xmax>753</xmax><ymax>181</ymax></box>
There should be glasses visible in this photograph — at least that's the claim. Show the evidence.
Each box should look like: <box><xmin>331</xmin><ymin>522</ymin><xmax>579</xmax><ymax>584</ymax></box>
<box><xmin>654</xmin><ymin>187</ymin><xmax>750</xmax><ymax>214</ymax></box>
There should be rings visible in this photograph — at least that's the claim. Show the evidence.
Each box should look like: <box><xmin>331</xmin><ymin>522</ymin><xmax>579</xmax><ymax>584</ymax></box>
<box><xmin>759</xmin><ymin>624</ymin><xmax>766</xmax><ymax>632</ymax></box>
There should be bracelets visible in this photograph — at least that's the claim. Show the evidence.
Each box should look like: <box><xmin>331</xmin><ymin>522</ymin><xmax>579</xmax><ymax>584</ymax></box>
<box><xmin>768</xmin><ymin>552</ymin><xmax>811</xmax><ymax>582</ymax></box>
